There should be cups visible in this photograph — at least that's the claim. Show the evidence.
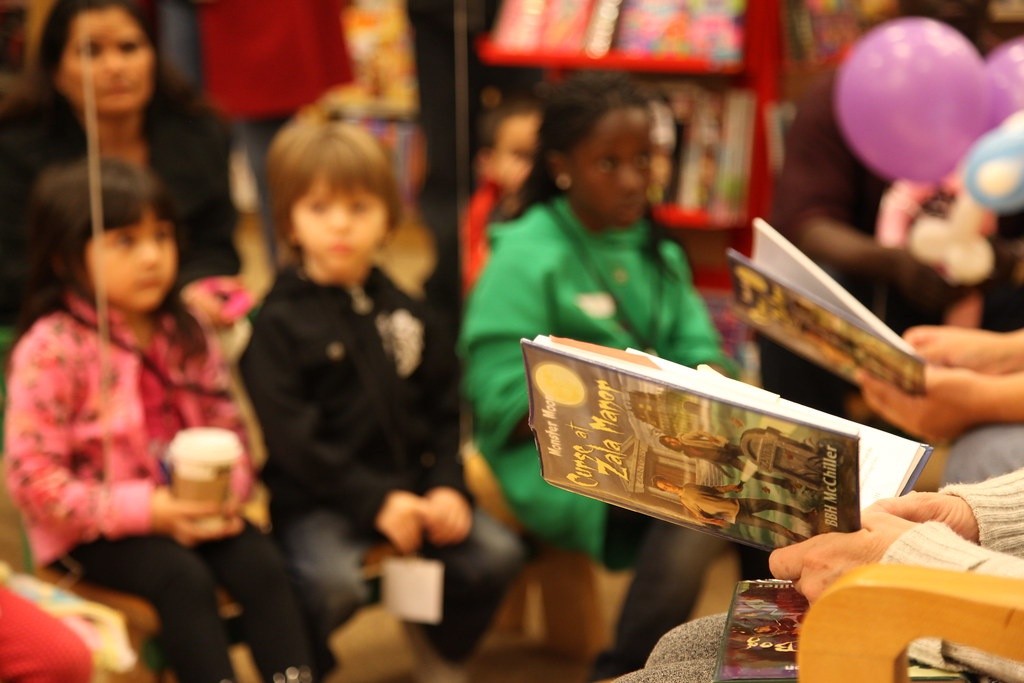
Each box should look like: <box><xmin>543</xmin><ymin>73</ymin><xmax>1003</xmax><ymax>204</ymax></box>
<box><xmin>166</xmin><ymin>427</ymin><xmax>244</xmax><ymax>530</ymax></box>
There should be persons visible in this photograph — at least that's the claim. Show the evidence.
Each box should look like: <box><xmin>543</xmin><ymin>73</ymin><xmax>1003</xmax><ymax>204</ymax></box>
<box><xmin>769</xmin><ymin>469</ymin><xmax>1024</xmax><ymax>683</ymax></box>
<box><xmin>857</xmin><ymin>323</ymin><xmax>1023</xmax><ymax>491</ymax></box>
<box><xmin>656</xmin><ymin>429</ymin><xmax>797</xmax><ymax>494</ymax></box>
<box><xmin>650</xmin><ymin>475</ymin><xmax>819</xmax><ymax>543</ymax></box>
<box><xmin>457</xmin><ymin>100</ymin><xmax>545</xmax><ymax>296</ymax></box>
<box><xmin>240</xmin><ymin>117</ymin><xmax>523</xmax><ymax>680</ymax></box>
<box><xmin>457</xmin><ymin>77</ymin><xmax>772</xmax><ymax>683</ymax></box>
<box><xmin>4</xmin><ymin>156</ymin><xmax>319</xmax><ymax>683</ymax></box>
<box><xmin>0</xmin><ymin>2</ymin><xmax>251</xmax><ymax>357</ymax></box>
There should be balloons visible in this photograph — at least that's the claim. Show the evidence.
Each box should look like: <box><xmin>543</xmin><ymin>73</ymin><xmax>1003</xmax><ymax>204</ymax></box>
<box><xmin>983</xmin><ymin>38</ymin><xmax>1023</xmax><ymax>132</ymax></box>
<box><xmin>831</xmin><ymin>19</ymin><xmax>991</xmax><ymax>188</ymax></box>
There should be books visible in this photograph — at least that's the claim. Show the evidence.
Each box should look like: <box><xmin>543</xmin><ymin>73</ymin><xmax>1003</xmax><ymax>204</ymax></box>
<box><xmin>711</xmin><ymin>580</ymin><xmax>973</xmax><ymax>683</ymax></box>
<box><xmin>725</xmin><ymin>215</ymin><xmax>931</xmax><ymax>399</ymax></box>
<box><xmin>521</xmin><ymin>328</ymin><xmax>933</xmax><ymax>552</ymax></box>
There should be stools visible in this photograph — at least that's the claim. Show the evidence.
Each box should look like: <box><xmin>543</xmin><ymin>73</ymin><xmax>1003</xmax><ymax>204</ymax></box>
<box><xmin>35</xmin><ymin>561</ymin><xmax>247</xmax><ymax>683</ymax></box>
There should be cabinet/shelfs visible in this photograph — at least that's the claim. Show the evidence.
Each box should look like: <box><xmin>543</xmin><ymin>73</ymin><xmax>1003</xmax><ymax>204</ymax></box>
<box><xmin>462</xmin><ymin>0</ymin><xmax>775</xmax><ymax>300</ymax></box>
